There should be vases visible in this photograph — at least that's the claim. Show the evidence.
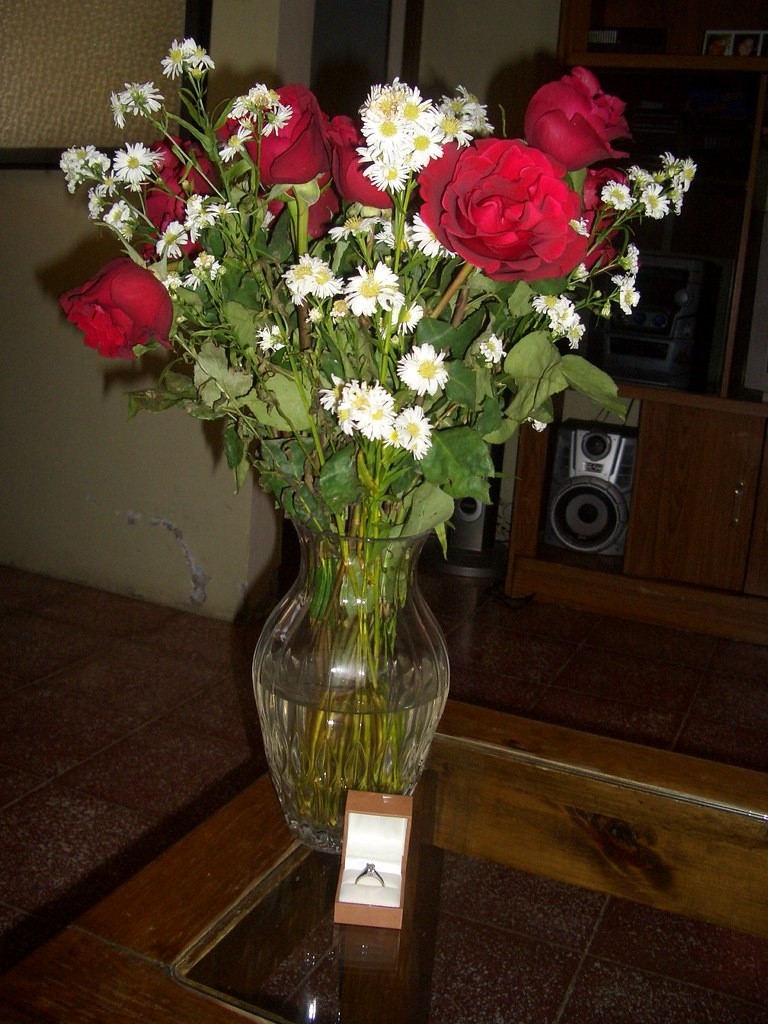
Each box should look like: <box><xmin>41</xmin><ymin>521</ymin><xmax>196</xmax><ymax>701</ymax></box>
<box><xmin>250</xmin><ymin>484</ymin><xmax>449</xmax><ymax>853</ymax></box>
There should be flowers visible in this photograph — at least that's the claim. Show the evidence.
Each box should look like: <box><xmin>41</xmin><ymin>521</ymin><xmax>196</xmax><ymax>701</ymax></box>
<box><xmin>57</xmin><ymin>34</ymin><xmax>698</xmax><ymax>821</ymax></box>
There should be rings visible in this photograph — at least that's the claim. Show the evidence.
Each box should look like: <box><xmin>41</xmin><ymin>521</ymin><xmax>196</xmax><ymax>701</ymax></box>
<box><xmin>355</xmin><ymin>864</ymin><xmax>385</xmax><ymax>886</ymax></box>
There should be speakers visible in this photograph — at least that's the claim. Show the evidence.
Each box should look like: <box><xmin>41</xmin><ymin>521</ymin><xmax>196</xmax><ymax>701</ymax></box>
<box><xmin>535</xmin><ymin>417</ymin><xmax>639</xmax><ymax>577</ymax></box>
<box><xmin>434</xmin><ymin>442</ymin><xmax>505</xmax><ymax>569</ymax></box>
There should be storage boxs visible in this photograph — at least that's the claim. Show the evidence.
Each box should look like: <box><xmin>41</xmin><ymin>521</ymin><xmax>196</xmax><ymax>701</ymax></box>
<box><xmin>334</xmin><ymin>789</ymin><xmax>413</xmax><ymax>930</ymax></box>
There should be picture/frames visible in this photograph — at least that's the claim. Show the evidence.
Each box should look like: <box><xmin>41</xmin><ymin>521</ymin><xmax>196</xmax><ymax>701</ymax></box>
<box><xmin>0</xmin><ymin>0</ymin><xmax>212</xmax><ymax>169</ymax></box>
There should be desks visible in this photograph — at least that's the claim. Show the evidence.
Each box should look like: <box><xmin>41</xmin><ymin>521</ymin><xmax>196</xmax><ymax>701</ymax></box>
<box><xmin>1</xmin><ymin>698</ymin><xmax>768</xmax><ymax>1024</ymax></box>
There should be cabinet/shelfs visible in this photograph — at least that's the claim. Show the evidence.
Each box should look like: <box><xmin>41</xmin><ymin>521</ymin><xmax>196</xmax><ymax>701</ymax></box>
<box><xmin>507</xmin><ymin>1</ymin><xmax>768</xmax><ymax>648</ymax></box>
